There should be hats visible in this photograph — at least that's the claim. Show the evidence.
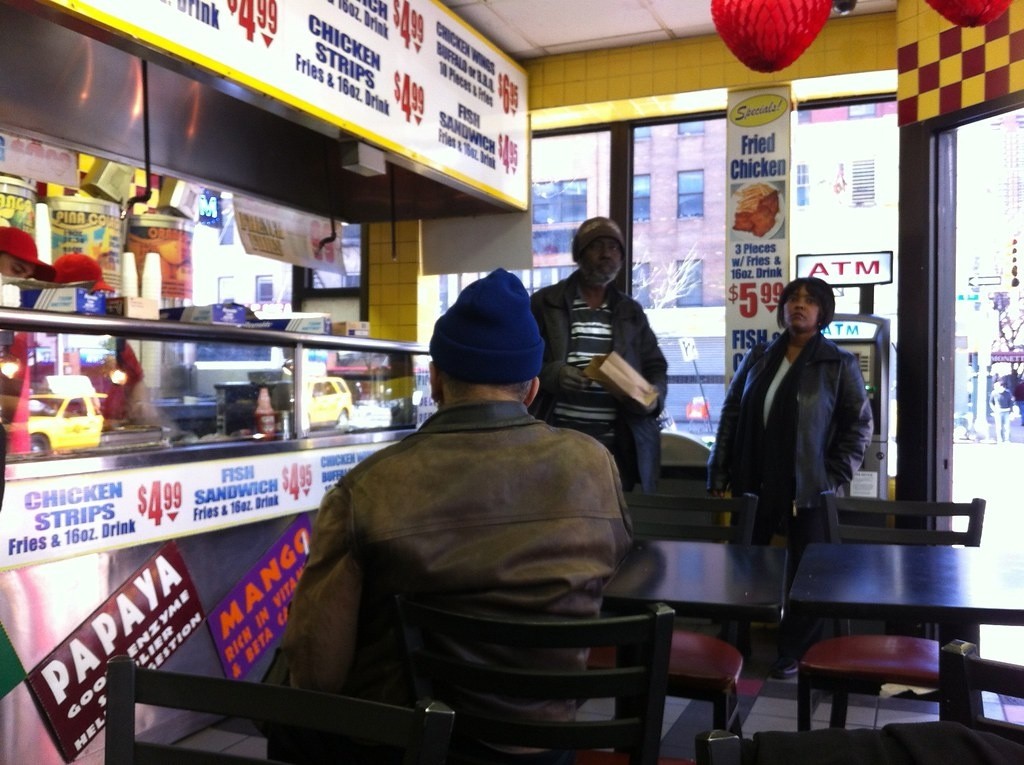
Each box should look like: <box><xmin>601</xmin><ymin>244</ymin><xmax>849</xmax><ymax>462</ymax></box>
<box><xmin>0</xmin><ymin>226</ymin><xmax>55</xmax><ymax>283</ymax></box>
<box><xmin>572</xmin><ymin>216</ymin><xmax>626</xmax><ymax>262</ymax></box>
<box><xmin>429</xmin><ymin>268</ymin><xmax>546</xmax><ymax>384</ymax></box>
<box><xmin>52</xmin><ymin>254</ymin><xmax>116</xmax><ymax>293</ymax></box>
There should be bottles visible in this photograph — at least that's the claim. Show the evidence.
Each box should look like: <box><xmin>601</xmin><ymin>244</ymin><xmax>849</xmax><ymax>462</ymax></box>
<box><xmin>255</xmin><ymin>387</ymin><xmax>277</xmax><ymax>440</ymax></box>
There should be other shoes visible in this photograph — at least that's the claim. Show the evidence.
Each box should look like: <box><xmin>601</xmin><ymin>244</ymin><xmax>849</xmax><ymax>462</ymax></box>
<box><xmin>769</xmin><ymin>654</ymin><xmax>800</xmax><ymax>679</ymax></box>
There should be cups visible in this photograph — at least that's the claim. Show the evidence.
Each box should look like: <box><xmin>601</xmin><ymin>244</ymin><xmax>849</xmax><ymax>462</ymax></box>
<box><xmin>121</xmin><ymin>251</ymin><xmax>163</xmax><ymax>309</ymax></box>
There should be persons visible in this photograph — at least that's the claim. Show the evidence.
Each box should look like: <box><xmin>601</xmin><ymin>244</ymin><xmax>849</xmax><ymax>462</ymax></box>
<box><xmin>706</xmin><ymin>278</ymin><xmax>875</xmax><ymax>679</ymax></box>
<box><xmin>0</xmin><ymin>224</ymin><xmax>158</xmax><ymax>433</ymax></box>
<box><xmin>527</xmin><ymin>217</ymin><xmax>669</xmax><ymax>493</ymax></box>
<box><xmin>282</xmin><ymin>268</ymin><xmax>636</xmax><ymax>765</ymax></box>
<box><xmin>989</xmin><ymin>382</ymin><xmax>1016</xmax><ymax>443</ymax></box>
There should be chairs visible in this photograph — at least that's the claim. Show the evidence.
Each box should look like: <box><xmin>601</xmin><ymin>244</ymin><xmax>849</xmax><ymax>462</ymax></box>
<box><xmin>939</xmin><ymin>645</ymin><xmax>1024</xmax><ymax>743</ymax></box>
<box><xmin>586</xmin><ymin>487</ymin><xmax>759</xmax><ymax>739</ymax></box>
<box><xmin>694</xmin><ymin>722</ymin><xmax>1024</xmax><ymax>765</ymax></box>
<box><xmin>106</xmin><ymin>655</ymin><xmax>454</xmax><ymax>765</ymax></box>
<box><xmin>796</xmin><ymin>492</ymin><xmax>985</xmax><ymax>731</ymax></box>
<box><xmin>381</xmin><ymin>588</ymin><xmax>675</xmax><ymax>765</ymax></box>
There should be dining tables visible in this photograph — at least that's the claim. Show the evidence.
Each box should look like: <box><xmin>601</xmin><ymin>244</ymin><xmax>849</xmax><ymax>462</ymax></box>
<box><xmin>601</xmin><ymin>540</ymin><xmax>1024</xmax><ymax>730</ymax></box>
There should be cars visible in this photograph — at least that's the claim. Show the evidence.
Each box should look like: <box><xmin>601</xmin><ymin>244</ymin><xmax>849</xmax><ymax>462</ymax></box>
<box><xmin>642</xmin><ymin>305</ymin><xmax>729</xmax><ymax>452</ymax></box>
<box><xmin>23</xmin><ymin>392</ymin><xmax>113</xmax><ymax>452</ymax></box>
<box><xmin>304</xmin><ymin>377</ymin><xmax>354</xmax><ymax>427</ymax></box>
